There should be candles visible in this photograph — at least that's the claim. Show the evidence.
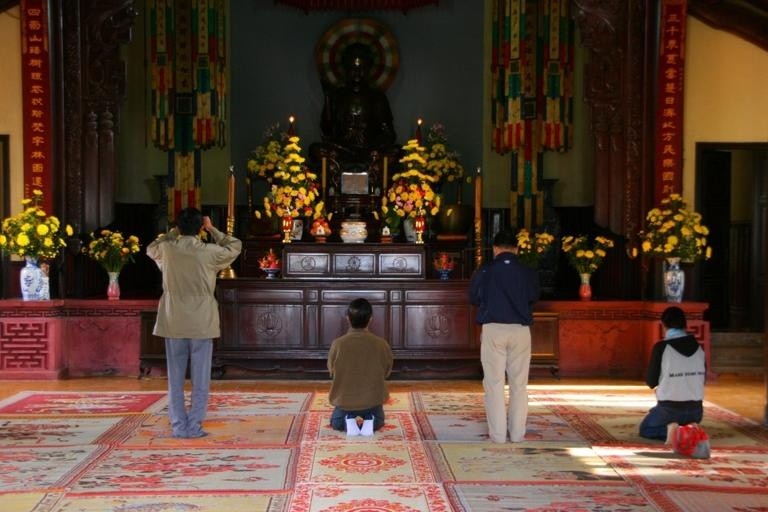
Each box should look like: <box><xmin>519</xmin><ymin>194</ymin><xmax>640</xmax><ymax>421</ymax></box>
<box><xmin>383</xmin><ymin>157</ymin><xmax>388</xmax><ymax>189</ymax></box>
<box><xmin>227</xmin><ymin>166</ymin><xmax>235</xmax><ymax>217</ymax></box>
<box><xmin>287</xmin><ymin>116</ymin><xmax>296</xmax><ymax>137</ymax></box>
<box><xmin>475</xmin><ymin>167</ymin><xmax>482</xmax><ymax>219</ymax></box>
<box><xmin>322</xmin><ymin>157</ymin><xmax>326</xmax><ymax>188</ymax></box>
<box><xmin>416</xmin><ymin>118</ymin><xmax>424</xmax><ymax>147</ymax></box>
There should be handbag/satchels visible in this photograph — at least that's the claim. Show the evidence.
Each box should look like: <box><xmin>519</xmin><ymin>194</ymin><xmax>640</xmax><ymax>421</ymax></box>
<box><xmin>672</xmin><ymin>425</ymin><xmax>710</xmax><ymax>458</ymax></box>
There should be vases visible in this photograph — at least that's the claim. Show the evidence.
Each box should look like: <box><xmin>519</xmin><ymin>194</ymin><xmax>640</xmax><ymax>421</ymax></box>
<box><xmin>663</xmin><ymin>257</ymin><xmax>685</xmax><ymax>302</ymax></box>
<box><xmin>577</xmin><ymin>272</ymin><xmax>593</xmax><ymax>302</ymax></box>
<box><xmin>107</xmin><ymin>271</ymin><xmax>121</xmax><ymax>300</ymax></box>
<box><xmin>413</xmin><ymin>216</ymin><xmax>426</xmax><ymax>243</ymax></box>
<box><xmin>279</xmin><ymin>217</ymin><xmax>294</xmax><ymax>244</ymax></box>
<box><xmin>19</xmin><ymin>256</ymin><xmax>44</xmax><ymax>302</ymax></box>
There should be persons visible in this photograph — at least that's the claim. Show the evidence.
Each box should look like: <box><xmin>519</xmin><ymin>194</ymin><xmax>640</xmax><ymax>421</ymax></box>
<box><xmin>328</xmin><ymin>298</ymin><xmax>397</xmax><ymax>440</ymax></box>
<box><xmin>469</xmin><ymin>230</ymin><xmax>542</xmax><ymax>447</ymax></box>
<box><xmin>639</xmin><ymin>307</ymin><xmax>708</xmax><ymax>445</ymax></box>
<box><xmin>309</xmin><ymin>53</ymin><xmax>401</xmax><ymax>192</ymax></box>
<box><xmin>146</xmin><ymin>205</ymin><xmax>244</xmax><ymax>441</ymax></box>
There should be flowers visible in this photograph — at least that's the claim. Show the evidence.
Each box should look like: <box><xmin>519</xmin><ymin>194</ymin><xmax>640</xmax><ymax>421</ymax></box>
<box><xmin>517</xmin><ymin>229</ymin><xmax>556</xmax><ymax>270</ymax></box>
<box><xmin>627</xmin><ymin>193</ymin><xmax>713</xmax><ymax>263</ymax></box>
<box><xmin>560</xmin><ymin>231</ymin><xmax>616</xmax><ymax>275</ymax></box>
<box><xmin>370</xmin><ymin>123</ymin><xmax>473</xmax><ymax>224</ymax></box>
<box><xmin>80</xmin><ymin>229</ymin><xmax>142</xmax><ymax>272</ymax></box>
<box><xmin>156</xmin><ymin>227</ymin><xmax>209</xmax><ymax>243</ymax></box>
<box><xmin>246</xmin><ymin>122</ymin><xmax>333</xmax><ymax>222</ymax></box>
<box><xmin>0</xmin><ymin>188</ymin><xmax>74</xmax><ymax>260</ymax></box>
